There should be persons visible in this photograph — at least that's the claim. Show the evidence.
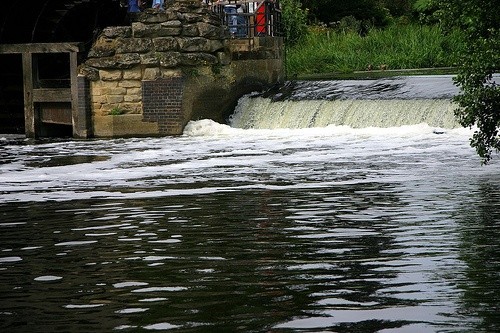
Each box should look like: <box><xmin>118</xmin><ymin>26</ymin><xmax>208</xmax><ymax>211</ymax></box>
<box><xmin>202</xmin><ymin>0</ymin><xmax>277</xmax><ymax>38</ymax></box>
<box><xmin>128</xmin><ymin>0</ymin><xmax>165</xmax><ymax>27</ymax></box>
